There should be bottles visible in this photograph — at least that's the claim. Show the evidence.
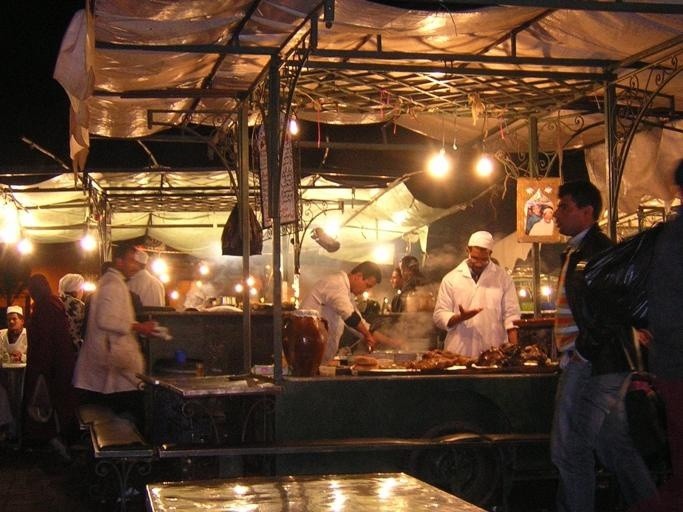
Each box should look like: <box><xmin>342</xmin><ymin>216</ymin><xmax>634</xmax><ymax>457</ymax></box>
<box><xmin>382</xmin><ymin>297</ymin><xmax>390</xmax><ymax>315</ymax></box>
<box><xmin>282</xmin><ymin>309</ymin><xmax>328</xmax><ymax>376</ymax></box>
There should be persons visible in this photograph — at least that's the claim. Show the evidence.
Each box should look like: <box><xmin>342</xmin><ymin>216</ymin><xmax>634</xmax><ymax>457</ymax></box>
<box><xmin>298</xmin><ymin>261</ymin><xmax>414</xmax><ymax>361</ymax></box>
<box><xmin>626</xmin><ymin>158</ymin><xmax>680</xmax><ymax>510</ymax></box>
<box><xmin>388</xmin><ymin>268</ymin><xmax>404</xmax><ymax>310</ymax></box>
<box><xmin>398</xmin><ymin>256</ymin><xmax>429</xmax><ymax>312</ymax></box>
<box><xmin>525</xmin><ymin>203</ymin><xmax>554</xmax><ymax>237</ymax></box>
<box><xmin>434</xmin><ymin>228</ymin><xmax>521</xmax><ymax>362</ymax></box>
<box><xmin>545</xmin><ymin>182</ymin><xmax>661</xmax><ymax>510</ymax></box>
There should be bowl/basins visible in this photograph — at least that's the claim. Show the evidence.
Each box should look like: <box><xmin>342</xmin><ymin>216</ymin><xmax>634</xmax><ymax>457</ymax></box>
<box><xmin>205</xmin><ymin>295</ymin><xmax>236</xmax><ymax>307</ymax></box>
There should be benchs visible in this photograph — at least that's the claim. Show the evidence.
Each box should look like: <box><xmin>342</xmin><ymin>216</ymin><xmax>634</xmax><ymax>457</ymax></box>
<box><xmin>73</xmin><ymin>404</ymin><xmax>117</xmax><ymax>432</ymax></box>
<box><xmin>151</xmin><ymin>426</ymin><xmax>552</xmax><ymax>511</ymax></box>
<box><xmin>87</xmin><ymin>417</ymin><xmax>154</xmax><ymax>512</ymax></box>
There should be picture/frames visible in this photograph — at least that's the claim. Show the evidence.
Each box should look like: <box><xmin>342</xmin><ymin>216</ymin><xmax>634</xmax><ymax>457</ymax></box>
<box><xmin>515</xmin><ymin>176</ymin><xmax>562</xmax><ymax>244</ymax></box>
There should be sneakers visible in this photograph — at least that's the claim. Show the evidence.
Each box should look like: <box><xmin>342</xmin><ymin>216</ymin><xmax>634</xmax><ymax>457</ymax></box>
<box><xmin>99</xmin><ymin>489</ymin><xmax>143</xmax><ymax>508</ymax></box>
<box><xmin>50</xmin><ymin>437</ymin><xmax>74</xmax><ymax>465</ymax></box>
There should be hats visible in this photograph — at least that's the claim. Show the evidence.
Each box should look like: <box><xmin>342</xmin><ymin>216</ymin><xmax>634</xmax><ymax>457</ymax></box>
<box><xmin>135</xmin><ymin>250</ymin><xmax>150</xmax><ymax>267</ymax></box>
<box><xmin>6</xmin><ymin>304</ymin><xmax>25</xmax><ymax>318</ymax></box>
<box><xmin>467</xmin><ymin>229</ymin><xmax>496</xmax><ymax>253</ymax></box>
<box><xmin>57</xmin><ymin>272</ymin><xmax>84</xmax><ymax>294</ymax></box>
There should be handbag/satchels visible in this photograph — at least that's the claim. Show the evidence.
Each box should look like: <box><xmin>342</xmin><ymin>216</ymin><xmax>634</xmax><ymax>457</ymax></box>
<box><xmin>612</xmin><ymin>370</ymin><xmax>673</xmax><ymax>510</ymax></box>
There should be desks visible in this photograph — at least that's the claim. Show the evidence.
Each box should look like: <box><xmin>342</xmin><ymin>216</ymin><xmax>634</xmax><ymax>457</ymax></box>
<box><xmin>140</xmin><ymin>466</ymin><xmax>491</xmax><ymax>512</ymax></box>
<box><xmin>135</xmin><ymin>361</ymin><xmax>282</xmax><ymax>475</ymax></box>
